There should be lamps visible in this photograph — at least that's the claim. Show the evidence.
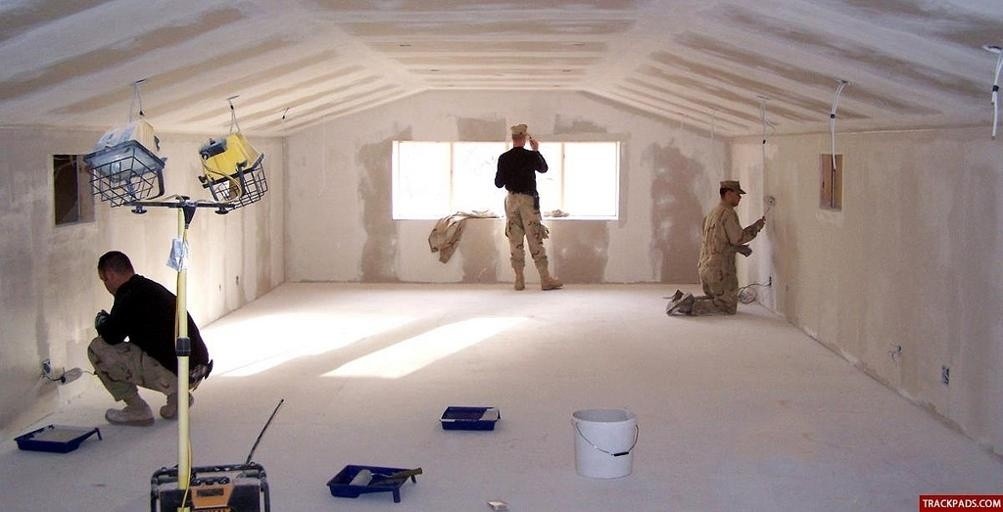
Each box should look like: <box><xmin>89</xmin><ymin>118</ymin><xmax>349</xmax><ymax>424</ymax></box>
<box><xmin>82</xmin><ymin>120</ymin><xmax>168</xmax><ymax>213</ymax></box>
<box><xmin>198</xmin><ymin>132</ymin><xmax>268</xmax><ymax>214</ymax></box>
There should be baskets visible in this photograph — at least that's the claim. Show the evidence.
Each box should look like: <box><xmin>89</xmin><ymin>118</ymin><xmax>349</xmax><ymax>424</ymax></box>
<box><xmin>202</xmin><ymin>153</ymin><xmax>268</xmax><ymax>211</ymax></box>
<box><xmin>82</xmin><ymin>140</ymin><xmax>165</xmax><ymax>207</ymax></box>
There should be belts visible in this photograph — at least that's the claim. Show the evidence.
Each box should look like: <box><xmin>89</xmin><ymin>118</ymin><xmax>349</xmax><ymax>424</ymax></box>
<box><xmin>189</xmin><ymin>374</ymin><xmax>206</xmax><ymax>384</ymax></box>
<box><xmin>516</xmin><ymin>191</ymin><xmax>538</xmax><ymax>195</ymax></box>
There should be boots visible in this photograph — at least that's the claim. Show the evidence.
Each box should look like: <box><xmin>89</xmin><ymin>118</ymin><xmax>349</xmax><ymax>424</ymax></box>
<box><xmin>105</xmin><ymin>392</ymin><xmax>155</xmax><ymax>427</ymax></box>
<box><xmin>514</xmin><ymin>264</ymin><xmax>525</xmax><ymax>290</ymax></box>
<box><xmin>536</xmin><ymin>258</ymin><xmax>563</xmax><ymax>290</ymax></box>
<box><xmin>160</xmin><ymin>391</ymin><xmax>194</xmax><ymax>420</ymax></box>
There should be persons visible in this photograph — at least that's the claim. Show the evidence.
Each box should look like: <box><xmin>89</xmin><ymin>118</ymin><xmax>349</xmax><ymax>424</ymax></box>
<box><xmin>494</xmin><ymin>124</ymin><xmax>563</xmax><ymax>290</ymax></box>
<box><xmin>666</xmin><ymin>180</ymin><xmax>765</xmax><ymax>315</ymax></box>
<box><xmin>87</xmin><ymin>250</ymin><xmax>213</xmax><ymax>426</ymax></box>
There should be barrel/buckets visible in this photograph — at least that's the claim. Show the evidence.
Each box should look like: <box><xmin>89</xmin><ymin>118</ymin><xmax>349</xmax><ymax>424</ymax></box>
<box><xmin>571</xmin><ymin>405</ymin><xmax>639</xmax><ymax>478</ymax></box>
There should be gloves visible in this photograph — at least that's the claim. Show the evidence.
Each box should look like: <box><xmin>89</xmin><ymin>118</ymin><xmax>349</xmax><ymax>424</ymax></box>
<box><xmin>539</xmin><ymin>224</ymin><xmax>549</xmax><ymax>240</ymax></box>
<box><xmin>95</xmin><ymin>310</ymin><xmax>110</xmax><ymax>329</ymax></box>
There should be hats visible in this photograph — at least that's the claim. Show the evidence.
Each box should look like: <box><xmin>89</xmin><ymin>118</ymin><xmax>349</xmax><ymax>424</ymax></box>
<box><xmin>511</xmin><ymin>124</ymin><xmax>528</xmax><ymax>135</ymax></box>
<box><xmin>720</xmin><ymin>181</ymin><xmax>745</xmax><ymax>194</ymax></box>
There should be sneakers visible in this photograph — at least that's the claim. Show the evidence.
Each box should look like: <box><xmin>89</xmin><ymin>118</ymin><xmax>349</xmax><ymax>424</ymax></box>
<box><xmin>667</xmin><ymin>293</ymin><xmax>694</xmax><ymax>316</ymax></box>
<box><xmin>671</xmin><ymin>289</ymin><xmax>683</xmax><ymax>300</ymax></box>
